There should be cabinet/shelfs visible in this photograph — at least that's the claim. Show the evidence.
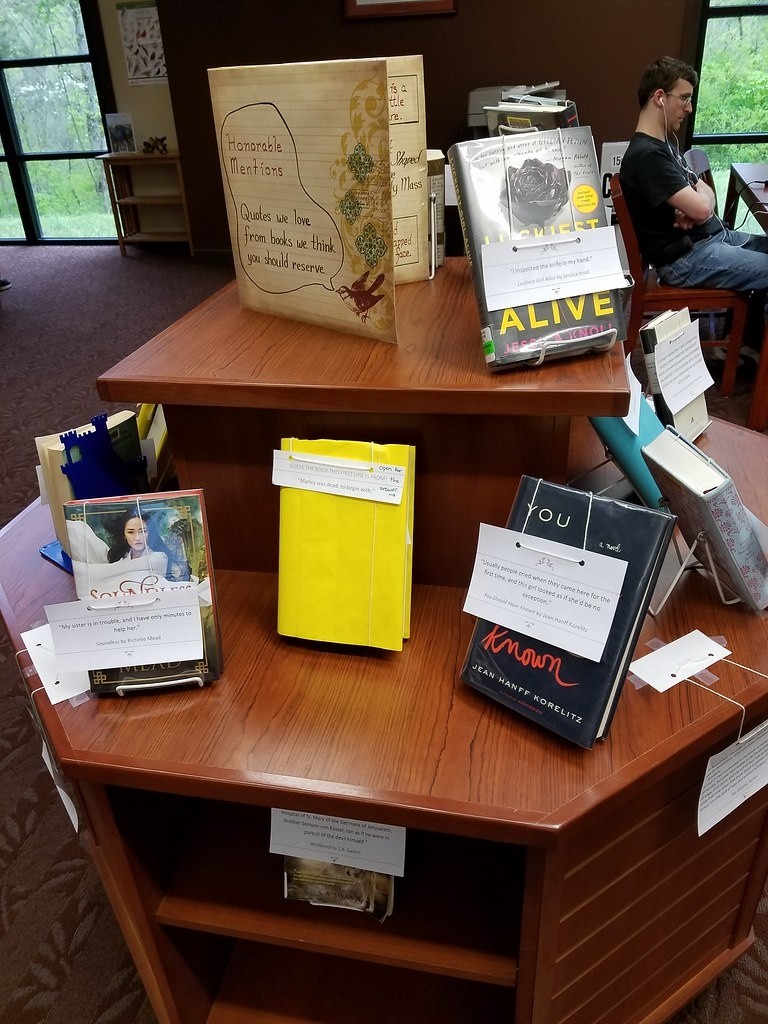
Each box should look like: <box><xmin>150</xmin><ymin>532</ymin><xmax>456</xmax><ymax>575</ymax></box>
<box><xmin>95</xmin><ymin>148</ymin><xmax>196</xmax><ymax>259</ymax></box>
<box><xmin>0</xmin><ymin>259</ymin><xmax>768</xmax><ymax>1023</ymax></box>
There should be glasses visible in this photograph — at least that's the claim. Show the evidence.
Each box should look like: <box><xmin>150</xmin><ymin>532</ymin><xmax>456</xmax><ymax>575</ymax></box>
<box><xmin>649</xmin><ymin>91</ymin><xmax>693</xmax><ymax>105</ymax></box>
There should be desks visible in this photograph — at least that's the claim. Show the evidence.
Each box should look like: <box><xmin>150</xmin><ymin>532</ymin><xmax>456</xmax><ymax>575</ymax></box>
<box><xmin>723</xmin><ymin>161</ymin><xmax>767</xmax><ymax>430</ymax></box>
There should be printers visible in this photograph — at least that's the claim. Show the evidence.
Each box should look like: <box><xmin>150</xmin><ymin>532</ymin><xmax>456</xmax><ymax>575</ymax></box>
<box><xmin>467</xmin><ymin>81</ymin><xmax>566</xmax><ymax>140</ymax></box>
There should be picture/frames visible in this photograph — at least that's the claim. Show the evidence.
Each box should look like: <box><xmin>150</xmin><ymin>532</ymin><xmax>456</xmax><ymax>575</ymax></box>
<box><xmin>337</xmin><ymin>0</ymin><xmax>460</xmax><ymax>25</ymax></box>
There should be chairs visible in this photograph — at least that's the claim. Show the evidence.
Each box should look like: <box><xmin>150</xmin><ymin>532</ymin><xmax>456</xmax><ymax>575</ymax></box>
<box><xmin>682</xmin><ymin>150</ymin><xmax>719</xmax><ymax>218</ymax></box>
<box><xmin>612</xmin><ymin>172</ymin><xmax>747</xmax><ymax>400</ymax></box>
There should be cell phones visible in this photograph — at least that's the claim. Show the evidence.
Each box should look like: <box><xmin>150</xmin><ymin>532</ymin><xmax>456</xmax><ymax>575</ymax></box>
<box><xmin>39</xmin><ymin>541</ymin><xmax>74</xmax><ymax>576</ymax></box>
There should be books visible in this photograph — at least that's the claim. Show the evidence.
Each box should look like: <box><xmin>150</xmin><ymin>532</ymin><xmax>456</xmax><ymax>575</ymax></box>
<box><xmin>458</xmin><ymin>304</ymin><xmax>767</xmax><ymax>753</ymax></box>
<box><xmin>448</xmin><ymin>78</ymin><xmax>637</xmax><ymax>373</ymax></box>
<box><xmin>270</xmin><ymin>434</ymin><xmax>415</xmax><ymax>653</ymax></box>
<box><xmin>34</xmin><ymin>403</ymin><xmax>227</xmax><ymax>698</ymax></box>
<box><xmin>206</xmin><ymin>53</ymin><xmax>445</xmax><ymax>346</ymax></box>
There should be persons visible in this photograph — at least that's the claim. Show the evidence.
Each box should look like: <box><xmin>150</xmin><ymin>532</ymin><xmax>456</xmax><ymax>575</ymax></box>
<box><xmin>616</xmin><ymin>56</ymin><xmax>768</xmax><ymax>390</ymax></box>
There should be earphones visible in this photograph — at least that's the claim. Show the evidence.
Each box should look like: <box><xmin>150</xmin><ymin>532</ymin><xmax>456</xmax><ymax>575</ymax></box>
<box><xmin>659</xmin><ymin>97</ymin><xmax>665</xmax><ymax>107</ymax></box>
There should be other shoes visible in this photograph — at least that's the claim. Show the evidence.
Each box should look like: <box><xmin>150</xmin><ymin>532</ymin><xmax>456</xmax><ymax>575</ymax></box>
<box><xmin>716</xmin><ymin>336</ymin><xmax>761</xmax><ymax>368</ymax></box>
